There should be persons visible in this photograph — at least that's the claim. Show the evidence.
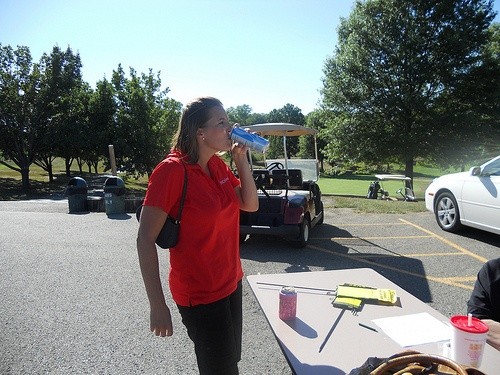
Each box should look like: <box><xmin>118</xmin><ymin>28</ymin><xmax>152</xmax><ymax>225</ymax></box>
<box><xmin>137</xmin><ymin>97</ymin><xmax>260</xmax><ymax>375</ymax></box>
<box><xmin>467</xmin><ymin>258</ymin><xmax>500</xmax><ymax>352</ymax></box>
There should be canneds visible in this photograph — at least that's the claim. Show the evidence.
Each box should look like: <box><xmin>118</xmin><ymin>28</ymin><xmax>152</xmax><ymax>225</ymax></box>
<box><xmin>279</xmin><ymin>285</ymin><xmax>298</xmax><ymax>321</ymax></box>
<box><xmin>231</xmin><ymin>126</ymin><xmax>271</xmax><ymax>154</ymax></box>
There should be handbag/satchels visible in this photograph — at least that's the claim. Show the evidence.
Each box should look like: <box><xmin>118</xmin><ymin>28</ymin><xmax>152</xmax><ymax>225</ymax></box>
<box><xmin>136</xmin><ymin>161</ymin><xmax>188</xmax><ymax>251</ymax></box>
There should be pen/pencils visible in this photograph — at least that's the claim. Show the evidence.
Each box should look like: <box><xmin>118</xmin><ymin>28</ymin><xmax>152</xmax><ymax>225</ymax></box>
<box><xmin>358</xmin><ymin>322</ymin><xmax>378</xmax><ymax>333</ymax></box>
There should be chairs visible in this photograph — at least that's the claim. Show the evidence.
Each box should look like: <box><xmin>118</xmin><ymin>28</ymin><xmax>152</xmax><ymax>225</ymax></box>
<box><xmin>273</xmin><ymin>169</ymin><xmax>304</xmax><ymax>186</ymax></box>
<box><xmin>250</xmin><ymin>168</ymin><xmax>270</xmax><ymax>185</ymax></box>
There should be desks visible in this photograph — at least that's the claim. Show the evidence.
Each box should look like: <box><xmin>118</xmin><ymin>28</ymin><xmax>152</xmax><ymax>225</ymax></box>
<box><xmin>247</xmin><ymin>266</ymin><xmax>500</xmax><ymax>375</ymax></box>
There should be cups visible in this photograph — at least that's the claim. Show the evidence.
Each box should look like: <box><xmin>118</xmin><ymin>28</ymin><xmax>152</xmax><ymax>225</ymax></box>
<box><xmin>450</xmin><ymin>315</ymin><xmax>490</xmax><ymax>371</ymax></box>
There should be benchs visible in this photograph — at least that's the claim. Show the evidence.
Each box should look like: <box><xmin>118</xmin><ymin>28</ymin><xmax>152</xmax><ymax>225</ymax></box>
<box><xmin>384</xmin><ymin>190</ymin><xmax>398</xmax><ymax>201</ymax></box>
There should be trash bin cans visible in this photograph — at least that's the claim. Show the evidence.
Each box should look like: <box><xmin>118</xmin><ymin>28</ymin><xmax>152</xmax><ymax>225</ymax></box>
<box><xmin>103</xmin><ymin>176</ymin><xmax>126</xmax><ymax>216</ymax></box>
<box><xmin>65</xmin><ymin>176</ymin><xmax>90</xmax><ymax>215</ymax></box>
